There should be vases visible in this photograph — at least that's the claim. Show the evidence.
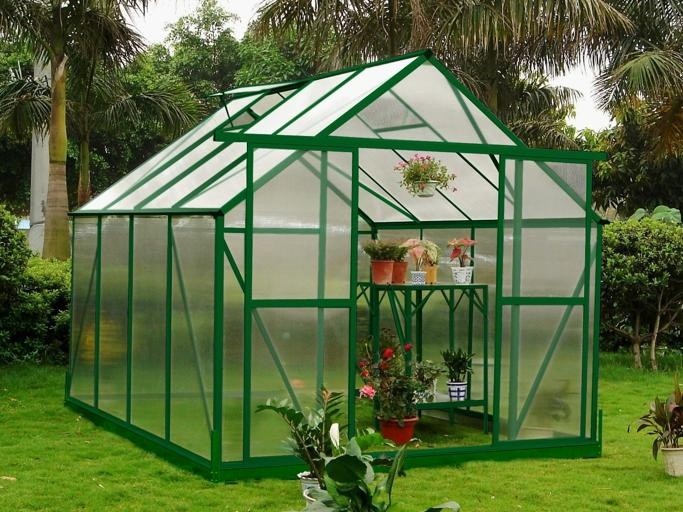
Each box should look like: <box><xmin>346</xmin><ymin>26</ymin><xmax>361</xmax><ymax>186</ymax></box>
<box><xmin>393</xmin><ymin>261</ymin><xmax>408</xmax><ymax>284</ymax></box>
<box><xmin>413</xmin><ymin>179</ymin><xmax>440</xmax><ymax>198</ymax></box>
<box><xmin>422</xmin><ymin>265</ymin><xmax>438</xmax><ymax>284</ymax></box>
<box><xmin>377</xmin><ymin>409</ymin><xmax>420</xmax><ymax>447</ymax></box>
<box><xmin>448</xmin><ymin>267</ymin><xmax>476</xmax><ymax>285</ymax></box>
<box><xmin>410</xmin><ymin>270</ymin><xmax>426</xmax><ymax>283</ymax></box>
<box><xmin>368</xmin><ymin>258</ymin><xmax>394</xmax><ymax>286</ymax></box>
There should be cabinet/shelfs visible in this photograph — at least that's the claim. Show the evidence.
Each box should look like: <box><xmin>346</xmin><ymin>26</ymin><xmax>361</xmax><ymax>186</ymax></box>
<box><xmin>351</xmin><ymin>279</ymin><xmax>489</xmax><ymax>435</ymax></box>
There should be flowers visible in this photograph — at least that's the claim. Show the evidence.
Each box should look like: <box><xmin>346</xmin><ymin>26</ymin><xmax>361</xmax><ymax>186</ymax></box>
<box><xmin>444</xmin><ymin>236</ymin><xmax>477</xmax><ymax>266</ymax></box>
<box><xmin>424</xmin><ymin>241</ymin><xmax>443</xmax><ymax>267</ymax></box>
<box><xmin>356</xmin><ymin>327</ymin><xmax>445</xmax><ymax>426</ymax></box>
<box><xmin>392</xmin><ymin>151</ymin><xmax>459</xmax><ymax>197</ymax></box>
<box><xmin>399</xmin><ymin>240</ymin><xmax>436</xmax><ymax>271</ymax></box>
<box><xmin>361</xmin><ymin>233</ymin><xmax>403</xmax><ymax>260</ymax></box>
<box><xmin>400</xmin><ymin>241</ymin><xmax>419</xmax><ymax>260</ymax></box>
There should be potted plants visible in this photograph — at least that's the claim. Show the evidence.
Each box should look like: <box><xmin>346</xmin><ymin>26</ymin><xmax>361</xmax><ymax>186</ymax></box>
<box><xmin>515</xmin><ymin>375</ymin><xmax>574</xmax><ymax>439</ymax></box>
<box><xmin>253</xmin><ymin>384</ymin><xmax>424</xmax><ymax>512</ymax></box>
<box><xmin>626</xmin><ymin>371</ymin><xmax>683</xmax><ymax>478</ymax></box>
<box><xmin>440</xmin><ymin>345</ymin><xmax>474</xmax><ymax>400</ymax></box>
